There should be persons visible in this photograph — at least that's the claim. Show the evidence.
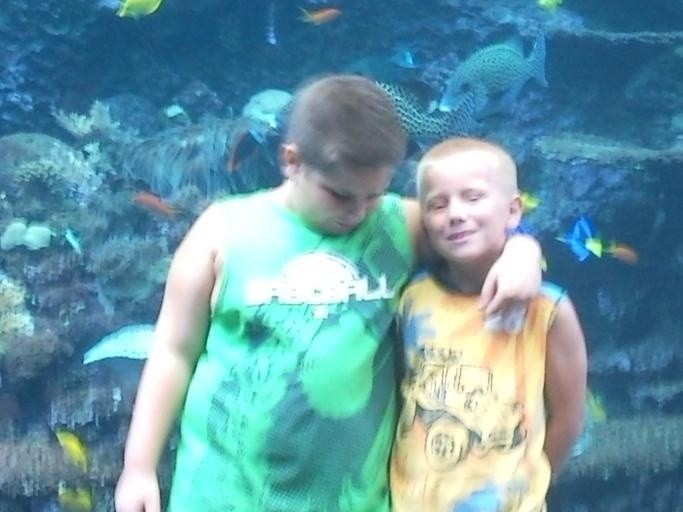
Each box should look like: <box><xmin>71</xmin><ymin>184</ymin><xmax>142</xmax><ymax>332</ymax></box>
<box><xmin>387</xmin><ymin>135</ymin><xmax>588</xmax><ymax>511</ymax></box>
<box><xmin>114</xmin><ymin>75</ymin><xmax>542</xmax><ymax>512</ymax></box>
